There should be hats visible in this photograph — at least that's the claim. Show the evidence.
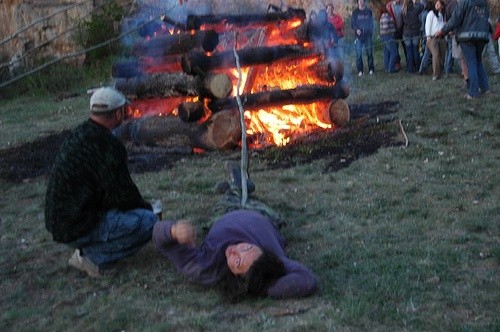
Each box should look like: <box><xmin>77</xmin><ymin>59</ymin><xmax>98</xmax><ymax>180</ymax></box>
<box><xmin>90</xmin><ymin>86</ymin><xmax>131</xmax><ymax>112</ymax></box>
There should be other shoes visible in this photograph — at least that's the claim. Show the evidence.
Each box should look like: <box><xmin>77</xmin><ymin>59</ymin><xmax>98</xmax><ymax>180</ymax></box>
<box><xmin>357</xmin><ymin>62</ymin><xmax>500</xmax><ymax>89</ymax></box>
<box><xmin>463</xmin><ymin>89</ymin><xmax>491</xmax><ymax>100</ymax></box>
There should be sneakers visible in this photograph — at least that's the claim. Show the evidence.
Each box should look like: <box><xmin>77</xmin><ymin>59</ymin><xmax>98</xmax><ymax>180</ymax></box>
<box><xmin>224</xmin><ymin>159</ymin><xmax>256</xmax><ymax>196</ymax></box>
<box><xmin>67</xmin><ymin>248</ymin><xmax>118</xmax><ymax>279</ymax></box>
<box><xmin>213</xmin><ymin>181</ymin><xmax>229</xmax><ymax>194</ymax></box>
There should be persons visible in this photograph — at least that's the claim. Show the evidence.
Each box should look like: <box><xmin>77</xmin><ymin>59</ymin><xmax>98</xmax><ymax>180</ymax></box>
<box><xmin>308</xmin><ymin>4</ymin><xmax>345</xmax><ymax>62</ymax></box>
<box><xmin>45</xmin><ymin>87</ymin><xmax>157</xmax><ymax>279</ymax></box>
<box><xmin>351</xmin><ymin>0</ymin><xmax>375</xmax><ymax>77</ymax></box>
<box><xmin>153</xmin><ymin>205</ymin><xmax>315</xmax><ymax>304</ymax></box>
<box><xmin>379</xmin><ymin>0</ymin><xmax>499</xmax><ymax>98</ymax></box>
